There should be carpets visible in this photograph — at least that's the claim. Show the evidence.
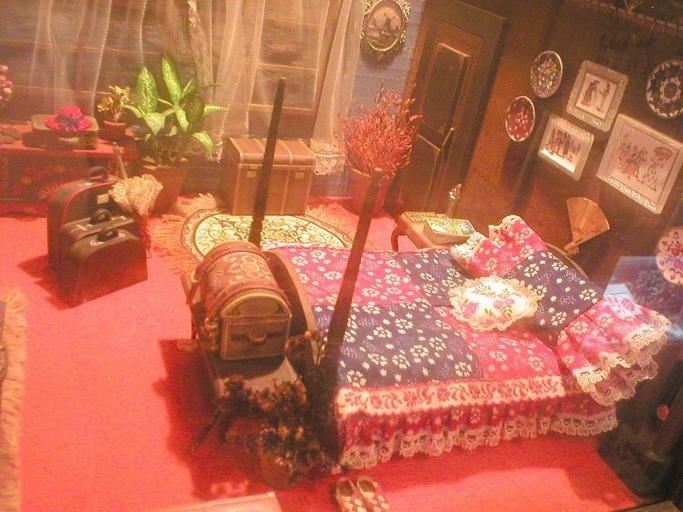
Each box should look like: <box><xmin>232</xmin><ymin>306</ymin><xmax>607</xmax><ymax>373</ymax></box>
<box><xmin>150</xmin><ymin>192</ymin><xmax>374</xmax><ymax>279</ymax></box>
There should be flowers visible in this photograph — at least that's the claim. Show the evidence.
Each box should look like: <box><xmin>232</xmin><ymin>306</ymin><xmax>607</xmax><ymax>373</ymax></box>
<box><xmin>0</xmin><ymin>65</ymin><xmax>14</xmax><ymax>109</ymax></box>
<box><xmin>333</xmin><ymin>82</ymin><xmax>424</xmax><ymax>177</ymax></box>
<box><xmin>98</xmin><ymin>85</ymin><xmax>133</xmax><ymax>121</ymax></box>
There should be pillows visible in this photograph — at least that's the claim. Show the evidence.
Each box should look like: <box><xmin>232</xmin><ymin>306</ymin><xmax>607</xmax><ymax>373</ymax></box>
<box><xmin>449</xmin><ymin>277</ymin><xmax>541</xmax><ymax>332</ymax></box>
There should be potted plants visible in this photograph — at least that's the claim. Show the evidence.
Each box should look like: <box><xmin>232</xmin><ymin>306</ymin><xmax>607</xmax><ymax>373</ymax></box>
<box><xmin>120</xmin><ymin>50</ymin><xmax>229</xmax><ymax>212</ymax></box>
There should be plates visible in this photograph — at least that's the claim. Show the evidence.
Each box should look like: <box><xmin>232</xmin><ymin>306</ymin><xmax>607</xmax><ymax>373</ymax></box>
<box><xmin>645</xmin><ymin>58</ymin><xmax>683</xmax><ymax>121</ymax></box>
<box><xmin>504</xmin><ymin>95</ymin><xmax>536</xmax><ymax>144</ymax></box>
<box><xmin>528</xmin><ymin>50</ymin><xmax>564</xmax><ymax>100</ymax></box>
<box><xmin>654</xmin><ymin>225</ymin><xmax>683</xmax><ymax>286</ymax></box>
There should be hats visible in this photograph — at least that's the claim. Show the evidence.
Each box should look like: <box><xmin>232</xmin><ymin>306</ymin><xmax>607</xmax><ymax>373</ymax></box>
<box><xmin>44</xmin><ymin>105</ymin><xmax>92</xmax><ymax>132</ymax></box>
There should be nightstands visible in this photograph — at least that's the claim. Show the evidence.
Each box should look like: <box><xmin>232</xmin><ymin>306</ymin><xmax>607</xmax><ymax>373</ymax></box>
<box><xmin>389</xmin><ymin>213</ymin><xmax>468</xmax><ymax>251</ymax></box>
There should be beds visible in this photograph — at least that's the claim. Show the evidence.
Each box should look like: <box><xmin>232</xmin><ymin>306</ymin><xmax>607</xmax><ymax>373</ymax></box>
<box><xmin>246</xmin><ymin>239</ymin><xmax>665</xmax><ymax>470</ymax></box>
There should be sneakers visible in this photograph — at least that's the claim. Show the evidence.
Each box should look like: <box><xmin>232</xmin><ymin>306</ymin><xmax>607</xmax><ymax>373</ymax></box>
<box><xmin>357</xmin><ymin>477</ymin><xmax>390</xmax><ymax>512</ymax></box>
<box><xmin>335</xmin><ymin>477</ymin><xmax>368</xmax><ymax>512</ymax></box>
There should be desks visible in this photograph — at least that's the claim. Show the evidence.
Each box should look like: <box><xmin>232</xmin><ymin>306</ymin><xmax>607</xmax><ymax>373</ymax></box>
<box><xmin>179</xmin><ymin>272</ymin><xmax>307</xmax><ymax>452</ymax></box>
<box><xmin>1</xmin><ymin>124</ymin><xmax>138</xmax><ymax>212</ymax></box>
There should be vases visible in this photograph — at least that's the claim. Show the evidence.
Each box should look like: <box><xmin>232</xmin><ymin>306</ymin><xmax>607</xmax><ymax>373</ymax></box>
<box><xmin>346</xmin><ymin>162</ymin><xmax>393</xmax><ymax>215</ymax></box>
<box><xmin>102</xmin><ymin>120</ymin><xmax>125</xmax><ymax>141</ymax></box>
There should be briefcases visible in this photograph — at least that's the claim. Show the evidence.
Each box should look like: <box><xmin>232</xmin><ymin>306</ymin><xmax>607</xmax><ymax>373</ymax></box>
<box><xmin>32</xmin><ymin>114</ymin><xmax>99</xmax><ymax>149</ymax></box>
<box><xmin>47</xmin><ymin>166</ymin><xmax>148</xmax><ymax>307</ymax></box>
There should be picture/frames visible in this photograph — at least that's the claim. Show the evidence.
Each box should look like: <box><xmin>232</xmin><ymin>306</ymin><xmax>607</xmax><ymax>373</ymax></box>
<box><xmin>357</xmin><ymin>0</ymin><xmax>410</xmax><ymax>60</ymax></box>
<box><xmin>537</xmin><ymin>112</ymin><xmax>595</xmax><ymax>181</ymax></box>
<box><xmin>566</xmin><ymin>58</ymin><xmax>629</xmax><ymax>133</ymax></box>
<box><xmin>595</xmin><ymin>114</ymin><xmax>683</xmax><ymax>214</ymax></box>
